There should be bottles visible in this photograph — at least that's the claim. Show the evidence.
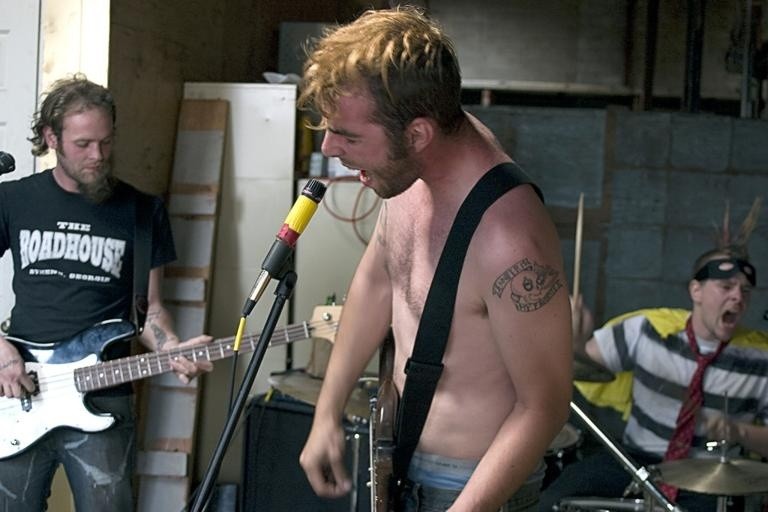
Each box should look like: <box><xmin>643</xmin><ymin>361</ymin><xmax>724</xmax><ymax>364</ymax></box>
<box><xmin>325</xmin><ymin>292</ymin><xmax>336</xmax><ymax>306</ymax></box>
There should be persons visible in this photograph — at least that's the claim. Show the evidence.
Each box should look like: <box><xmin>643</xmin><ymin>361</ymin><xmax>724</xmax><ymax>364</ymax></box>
<box><xmin>540</xmin><ymin>196</ymin><xmax>767</xmax><ymax>512</ymax></box>
<box><xmin>293</xmin><ymin>3</ymin><xmax>575</xmax><ymax>511</ymax></box>
<box><xmin>0</xmin><ymin>71</ymin><xmax>215</xmax><ymax>512</ymax></box>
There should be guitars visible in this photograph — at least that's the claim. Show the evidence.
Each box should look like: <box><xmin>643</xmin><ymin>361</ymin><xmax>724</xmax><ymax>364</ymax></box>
<box><xmin>0</xmin><ymin>305</ymin><xmax>349</xmax><ymax>460</ymax></box>
<box><xmin>369</xmin><ymin>330</ymin><xmax>398</xmax><ymax>512</ymax></box>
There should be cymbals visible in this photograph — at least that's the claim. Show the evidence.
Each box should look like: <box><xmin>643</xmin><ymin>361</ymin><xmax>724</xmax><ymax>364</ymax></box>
<box><xmin>263</xmin><ymin>370</ymin><xmax>381</xmax><ymax>422</ymax></box>
<box><xmin>645</xmin><ymin>457</ymin><xmax>767</xmax><ymax>494</ymax></box>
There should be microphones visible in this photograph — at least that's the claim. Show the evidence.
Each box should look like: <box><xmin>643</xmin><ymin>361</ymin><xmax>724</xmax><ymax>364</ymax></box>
<box><xmin>242</xmin><ymin>177</ymin><xmax>327</xmax><ymax>317</ymax></box>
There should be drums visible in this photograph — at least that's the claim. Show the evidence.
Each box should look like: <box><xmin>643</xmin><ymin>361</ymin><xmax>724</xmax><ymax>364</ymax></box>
<box><xmin>544</xmin><ymin>425</ymin><xmax>584</xmax><ymax>484</ymax></box>
<box><xmin>552</xmin><ymin>495</ymin><xmax>674</xmax><ymax>511</ymax></box>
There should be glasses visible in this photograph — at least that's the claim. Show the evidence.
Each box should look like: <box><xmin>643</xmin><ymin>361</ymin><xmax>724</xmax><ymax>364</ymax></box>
<box><xmin>693</xmin><ymin>257</ymin><xmax>757</xmax><ymax>287</ymax></box>
